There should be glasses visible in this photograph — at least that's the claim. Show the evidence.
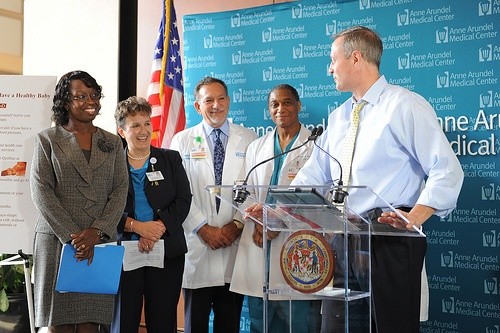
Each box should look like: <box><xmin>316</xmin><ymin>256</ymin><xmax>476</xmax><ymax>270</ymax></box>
<box><xmin>70</xmin><ymin>92</ymin><xmax>101</xmax><ymax>100</ymax></box>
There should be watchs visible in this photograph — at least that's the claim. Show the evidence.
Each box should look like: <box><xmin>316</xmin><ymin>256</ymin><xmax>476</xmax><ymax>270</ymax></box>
<box><xmin>234</xmin><ymin>221</ymin><xmax>243</xmax><ymax>229</ymax></box>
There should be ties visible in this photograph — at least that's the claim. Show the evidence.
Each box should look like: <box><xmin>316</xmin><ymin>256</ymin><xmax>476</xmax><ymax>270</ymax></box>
<box><xmin>334</xmin><ymin>99</ymin><xmax>369</xmax><ymax>217</ymax></box>
<box><xmin>214</xmin><ymin>129</ymin><xmax>225</xmax><ymax>214</ymax></box>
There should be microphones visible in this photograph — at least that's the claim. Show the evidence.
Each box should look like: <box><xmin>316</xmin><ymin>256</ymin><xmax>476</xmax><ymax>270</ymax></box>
<box><xmin>235</xmin><ymin>126</ymin><xmax>349</xmax><ymax>204</ymax></box>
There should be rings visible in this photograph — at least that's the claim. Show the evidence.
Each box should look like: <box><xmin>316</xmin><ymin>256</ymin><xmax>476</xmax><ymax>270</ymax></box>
<box><xmin>81</xmin><ymin>244</ymin><xmax>86</xmax><ymax>249</ymax></box>
<box><xmin>145</xmin><ymin>246</ymin><xmax>148</xmax><ymax>248</ymax></box>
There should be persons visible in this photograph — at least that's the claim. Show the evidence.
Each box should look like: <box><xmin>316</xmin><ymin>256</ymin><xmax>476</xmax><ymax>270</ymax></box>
<box><xmin>228</xmin><ymin>85</ymin><xmax>322</xmax><ymax>333</ymax></box>
<box><xmin>243</xmin><ymin>27</ymin><xmax>465</xmax><ymax>333</ymax></box>
<box><xmin>29</xmin><ymin>70</ymin><xmax>130</xmax><ymax>333</ymax></box>
<box><xmin>156</xmin><ymin>172</ymin><xmax>161</xmax><ymax>176</ymax></box>
<box><xmin>113</xmin><ymin>96</ymin><xmax>193</xmax><ymax>333</ymax></box>
<box><xmin>168</xmin><ymin>78</ymin><xmax>259</xmax><ymax>333</ymax></box>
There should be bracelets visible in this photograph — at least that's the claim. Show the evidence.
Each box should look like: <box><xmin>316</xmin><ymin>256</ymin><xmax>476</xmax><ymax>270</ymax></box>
<box><xmin>129</xmin><ymin>219</ymin><xmax>134</xmax><ymax>232</ymax></box>
<box><xmin>95</xmin><ymin>228</ymin><xmax>106</xmax><ymax>240</ymax></box>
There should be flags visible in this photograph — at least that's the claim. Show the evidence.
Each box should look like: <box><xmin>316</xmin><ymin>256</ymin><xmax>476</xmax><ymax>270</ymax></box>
<box><xmin>146</xmin><ymin>0</ymin><xmax>186</xmax><ymax>150</ymax></box>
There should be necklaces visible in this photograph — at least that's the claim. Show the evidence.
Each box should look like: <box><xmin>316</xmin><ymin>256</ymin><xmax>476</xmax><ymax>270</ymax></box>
<box><xmin>127</xmin><ymin>151</ymin><xmax>150</xmax><ymax>160</ymax></box>
